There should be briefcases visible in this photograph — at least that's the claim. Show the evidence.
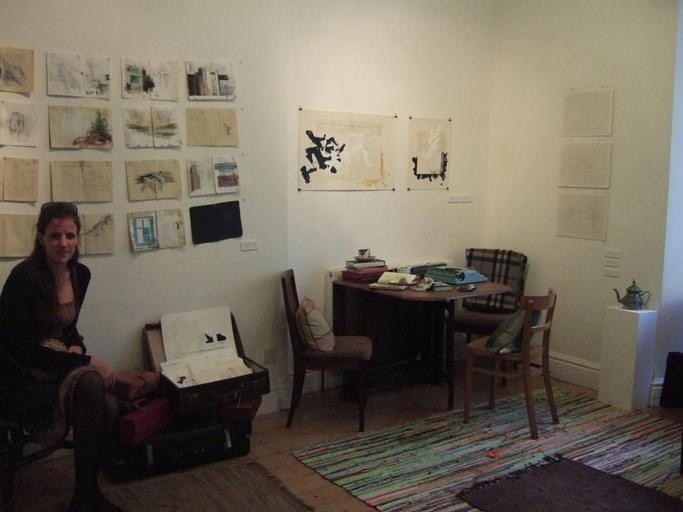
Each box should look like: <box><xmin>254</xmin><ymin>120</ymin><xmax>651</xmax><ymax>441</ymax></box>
<box><xmin>120</xmin><ymin>396</ymin><xmax>169</xmax><ymax>444</ymax></box>
<box><xmin>145</xmin><ymin>313</ymin><xmax>270</xmax><ymax>411</ymax></box>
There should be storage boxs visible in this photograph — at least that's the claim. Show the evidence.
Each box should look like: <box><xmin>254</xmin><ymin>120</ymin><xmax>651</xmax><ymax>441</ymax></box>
<box><xmin>113</xmin><ymin>312</ymin><xmax>269</xmax><ymax>445</ymax></box>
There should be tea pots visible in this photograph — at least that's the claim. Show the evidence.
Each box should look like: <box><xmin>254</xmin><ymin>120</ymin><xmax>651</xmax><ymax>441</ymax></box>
<box><xmin>612</xmin><ymin>278</ymin><xmax>651</xmax><ymax>309</ymax></box>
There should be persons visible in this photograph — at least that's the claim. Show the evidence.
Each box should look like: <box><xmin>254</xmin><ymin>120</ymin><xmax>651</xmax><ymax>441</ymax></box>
<box><xmin>0</xmin><ymin>200</ymin><xmax>123</xmax><ymax>511</ymax></box>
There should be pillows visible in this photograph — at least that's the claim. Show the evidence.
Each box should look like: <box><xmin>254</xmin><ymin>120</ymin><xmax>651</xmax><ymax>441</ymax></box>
<box><xmin>487</xmin><ymin>301</ymin><xmax>542</xmax><ymax>359</ymax></box>
<box><xmin>295</xmin><ymin>295</ymin><xmax>336</xmax><ymax>353</ymax></box>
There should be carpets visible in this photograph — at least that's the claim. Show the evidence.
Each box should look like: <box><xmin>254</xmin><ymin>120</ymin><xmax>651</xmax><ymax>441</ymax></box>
<box><xmin>455</xmin><ymin>452</ymin><xmax>681</xmax><ymax>511</ymax></box>
<box><xmin>290</xmin><ymin>386</ymin><xmax>681</xmax><ymax>512</ymax></box>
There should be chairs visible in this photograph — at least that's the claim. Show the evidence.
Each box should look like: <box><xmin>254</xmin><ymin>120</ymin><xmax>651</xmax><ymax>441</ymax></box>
<box><xmin>444</xmin><ymin>247</ymin><xmax>557</xmax><ymax>441</ymax></box>
<box><xmin>280</xmin><ymin>268</ymin><xmax>373</xmax><ymax>432</ymax></box>
<box><xmin>0</xmin><ymin>416</ymin><xmax>76</xmax><ymax>501</ymax></box>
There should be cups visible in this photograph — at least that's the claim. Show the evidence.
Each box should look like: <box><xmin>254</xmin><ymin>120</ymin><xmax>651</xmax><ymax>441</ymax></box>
<box><xmin>358</xmin><ymin>248</ymin><xmax>370</xmax><ymax>257</ymax></box>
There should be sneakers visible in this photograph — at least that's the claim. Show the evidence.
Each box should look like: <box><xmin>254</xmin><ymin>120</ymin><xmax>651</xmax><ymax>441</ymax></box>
<box><xmin>71</xmin><ymin>494</ymin><xmax>122</xmax><ymax>511</ymax></box>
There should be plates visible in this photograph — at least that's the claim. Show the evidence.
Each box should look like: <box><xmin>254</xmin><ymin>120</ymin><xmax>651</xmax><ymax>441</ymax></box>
<box><xmin>354</xmin><ymin>255</ymin><xmax>376</xmax><ymax>261</ymax></box>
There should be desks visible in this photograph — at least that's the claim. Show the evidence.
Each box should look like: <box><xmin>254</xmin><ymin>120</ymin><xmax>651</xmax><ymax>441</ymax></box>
<box><xmin>330</xmin><ymin>273</ymin><xmax>512</xmax><ymax>414</ymax></box>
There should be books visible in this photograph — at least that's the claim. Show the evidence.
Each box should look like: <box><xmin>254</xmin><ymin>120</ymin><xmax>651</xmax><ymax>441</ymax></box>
<box><xmin>368</xmin><ymin>271</ymin><xmax>416</xmax><ymax>291</ymax></box>
